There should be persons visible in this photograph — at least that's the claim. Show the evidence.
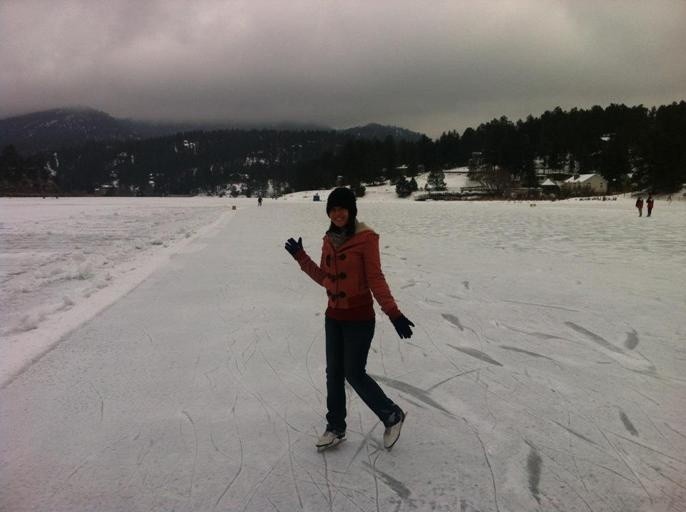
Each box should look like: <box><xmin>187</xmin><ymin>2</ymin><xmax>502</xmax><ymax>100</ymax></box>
<box><xmin>646</xmin><ymin>192</ymin><xmax>653</xmax><ymax>217</ymax></box>
<box><xmin>636</xmin><ymin>193</ymin><xmax>643</xmax><ymax>217</ymax></box>
<box><xmin>285</xmin><ymin>188</ymin><xmax>416</xmax><ymax>448</ymax></box>
<box><xmin>258</xmin><ymin>196</ymin><xmax>263</xmax><ymax>206</ymax></box>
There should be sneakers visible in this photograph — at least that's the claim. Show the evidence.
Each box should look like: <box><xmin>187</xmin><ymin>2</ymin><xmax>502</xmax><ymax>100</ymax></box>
<box><xmin>383</xmin><ymin>409</ymin><xmax>404</xmax><ymax>448</ymax></box>
<box><xmin>315</xmin><ymin>430</ymin><xmax>345</xmax><ymax>446</ymax></box>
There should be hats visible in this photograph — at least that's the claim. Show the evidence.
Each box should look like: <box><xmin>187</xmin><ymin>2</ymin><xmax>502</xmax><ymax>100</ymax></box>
<box><xmin>327</xmin><ymin>188</ymin><xmax>357</xmax><ymax>214</ymax></box>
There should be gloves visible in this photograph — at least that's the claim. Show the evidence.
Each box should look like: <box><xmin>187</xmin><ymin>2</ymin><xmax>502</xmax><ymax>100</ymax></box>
<box><xmin>392</xmin><ymin>314</ymin><xmax>415</xmax><ymax>339</ymax></box>
<box><xmin>284</xmin><ymin>237</ymin><xmax>305</xmax><ymax>259</ymax></box>
